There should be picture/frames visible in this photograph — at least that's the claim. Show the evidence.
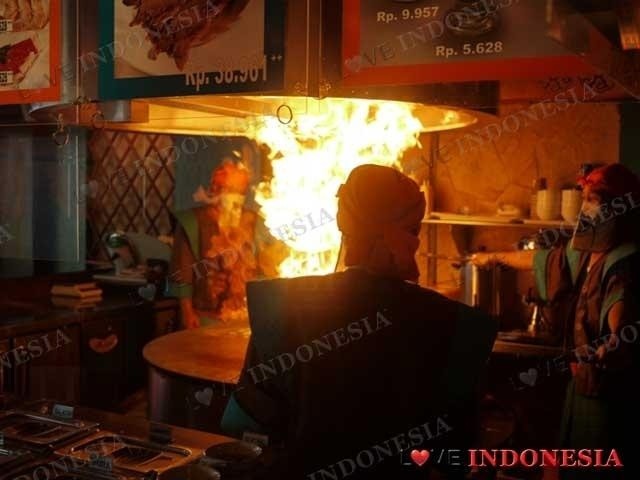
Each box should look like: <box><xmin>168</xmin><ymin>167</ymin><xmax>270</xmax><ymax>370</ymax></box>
<box><xmin>0</xmin><ymin>0</ymin><xmax>599</xmax><ymax>105</ymax></box>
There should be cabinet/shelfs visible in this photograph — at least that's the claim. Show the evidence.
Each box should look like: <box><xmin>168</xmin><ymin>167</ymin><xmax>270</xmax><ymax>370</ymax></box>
<box><xmin>422</xmin><ymin>211</ymin><xmax>581</xmax><ymax>358</ymax></box>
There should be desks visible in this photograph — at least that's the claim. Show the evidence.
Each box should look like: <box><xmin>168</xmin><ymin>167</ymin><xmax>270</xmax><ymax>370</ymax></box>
<box><xmin>141</xmin><ymin>319</ymin><xmax>252</xmax><ymax>435</ymax></box>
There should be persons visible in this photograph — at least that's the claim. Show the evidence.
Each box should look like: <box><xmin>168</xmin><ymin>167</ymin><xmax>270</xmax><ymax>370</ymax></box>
<box><xmin>221</xmin><ymin>165</ymin><xmax>498</xmax><ymax>480</ymax></box>
<box><xmin>469</xmin><ymin>164</ymin><xmax>640</xmax><ymax>480</ymax></box>
<box><xmin>168</xmin><ymin>159</ymin><xmax>276</xmax><ymax>330</ymax></box>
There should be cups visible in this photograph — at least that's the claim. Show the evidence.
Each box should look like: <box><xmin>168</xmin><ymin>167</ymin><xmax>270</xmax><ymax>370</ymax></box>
<box><xmin>202</xmin><ymin>442</ymin><xmax>264</xmax><ymax>479</ymax></box>
<box><xmin>535</xmin><ymin>188</ymin><xmax>583</xmax><ymax>225</ymax></box>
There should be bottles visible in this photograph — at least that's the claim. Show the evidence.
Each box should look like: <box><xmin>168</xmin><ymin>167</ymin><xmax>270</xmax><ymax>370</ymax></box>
<box><xmin>526</xmin><ymin>300</ymin><xmax>549</xmax><ymax>340</ymax></box>
